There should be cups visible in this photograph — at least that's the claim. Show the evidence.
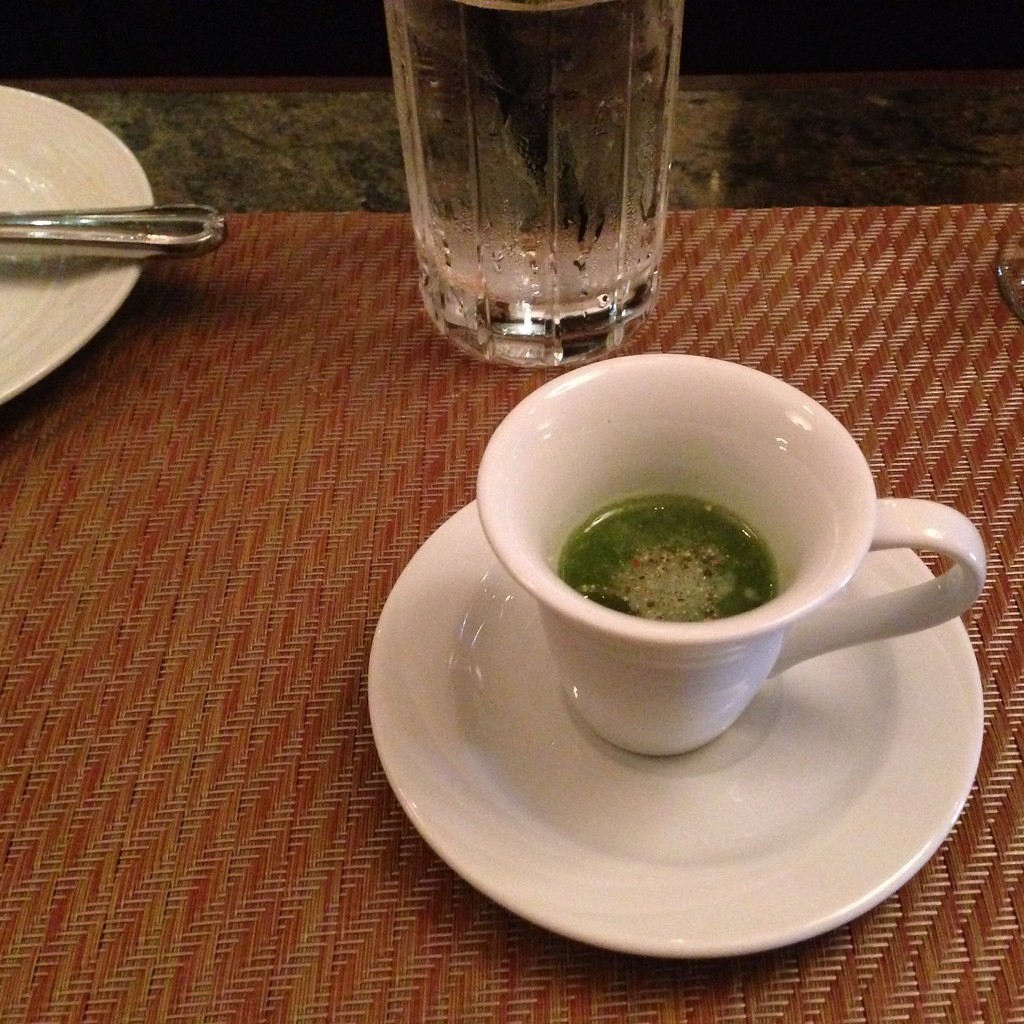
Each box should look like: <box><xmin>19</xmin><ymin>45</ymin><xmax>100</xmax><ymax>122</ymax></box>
<box><xmin>476</xmin><ymin>354</ymin><xmax>984</xmax><ymax>756</ymax></box>
<box><xmin>386</xmin><ymin>0</ymin><xmax>683</xmax><ymax>366</ymax></box>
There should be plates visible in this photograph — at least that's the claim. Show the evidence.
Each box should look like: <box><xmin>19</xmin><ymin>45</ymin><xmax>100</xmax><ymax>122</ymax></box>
<box><xmin>367</xmin><ymin>496</ymin><xmax>984</xmax><ymax>958</ymax></box>
<box><xmin>0</xmin><ymin>87</ymin><xmax>154</xmax><ymax>405</ymax></box>
